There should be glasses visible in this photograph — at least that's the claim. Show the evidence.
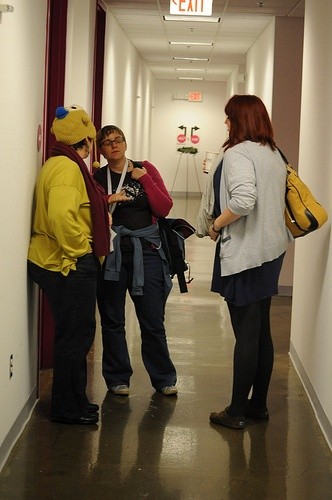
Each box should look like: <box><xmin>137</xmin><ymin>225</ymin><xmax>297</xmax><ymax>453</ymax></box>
<box><xmin>101</xmin><ymin>138</ymin><xmax>125</xmax><ymax>147</ymax></box>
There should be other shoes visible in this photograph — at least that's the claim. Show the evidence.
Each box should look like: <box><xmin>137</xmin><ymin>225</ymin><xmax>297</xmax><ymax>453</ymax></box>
<box><xmin>162</xmin><ymin>385</ymin><xmax>179</xmax><ymax>394</ymax></box>
<box><xmin>110</xmin><ymin>385</ymin><xmax>129</xmax><ymax>395</ymax></box>
<box><xmin>248</xmin><ymin>410</ymin><xmax>270</xmax><ymax>419</ymax></box>
<box><xmin>79</xmin><ymin>403</ymin><xmax>99</xmax><ymax>410</ymax></box>
<box><xmin>58</xmin><ymin>408</ymin><xmax>100</xmax><ymax>424</ymax></box>
<box><xmin>209</xmin><ymin>412</ymin><xmax>247</xmax><ymax>429</ymax></box>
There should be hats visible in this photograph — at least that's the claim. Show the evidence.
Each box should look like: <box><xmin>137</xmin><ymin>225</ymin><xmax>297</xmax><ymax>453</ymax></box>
<box><xmin>51</xmin><ymin>105</ymin><xmax>96</xmax><ymax>144</ymax></box>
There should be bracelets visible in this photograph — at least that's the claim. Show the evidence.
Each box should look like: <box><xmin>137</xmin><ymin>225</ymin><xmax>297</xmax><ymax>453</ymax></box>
<box><xmin>212</xmin><ymin>220</ymin><xmax>222</xmax><ymax>233</ymax></box>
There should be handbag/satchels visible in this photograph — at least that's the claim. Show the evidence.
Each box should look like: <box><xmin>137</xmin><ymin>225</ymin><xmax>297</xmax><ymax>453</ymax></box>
<box><xmin>273</xmin><ymin>144</ymin><xmax>329</xmax><ymax>238</ymax></box>
<box><xmin>160</xmin><ymin>218</ymin><xmax>196</xmax><ymax>273</ymax></box>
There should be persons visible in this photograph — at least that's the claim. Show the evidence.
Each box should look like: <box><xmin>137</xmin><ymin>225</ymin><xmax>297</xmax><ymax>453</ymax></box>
<box><xmin>196</xmin><ymin>94</ymin><xmax>289</xmax><ymax>430</ymax></box>
<box><xmin>89</xmin><ymin>124</ymin><xmax>179</xmax><ymax>395</ymax></box>
<box><xmin>27</xmin><ymin>104</ymin><xmax>112</xmax><ymax>424</ymax></box>
